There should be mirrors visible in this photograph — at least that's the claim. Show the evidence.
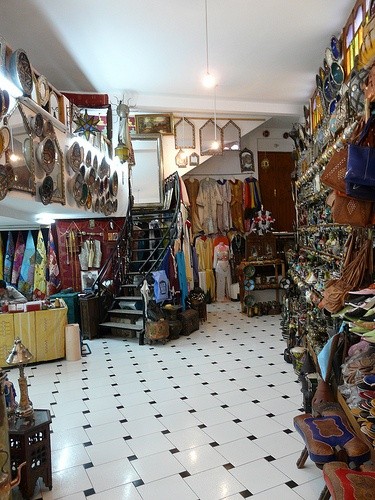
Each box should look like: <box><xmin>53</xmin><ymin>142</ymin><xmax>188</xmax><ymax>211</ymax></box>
<box><xmin>199</xmin><ymin>118</ymin><xmax>223</xmax><ymax>156</ymax></box>
<box><xmin>174</xmin><ymin>116</ymin><xmax>196</xmax><ymax>149</ymax></box>
<box><xmin>43</xmin><ymin>119</ymin><xmax>66</xmax><ymax>205</ymax></box>
<box><xmin>4</xmin><ymin>101</ymin><xmax>36</xmax><ymax>195</ymax></box>
<box><xmin>222</xmin><ymin>119</ymin><xmax>241</xmax><ymax>151</ymax></box>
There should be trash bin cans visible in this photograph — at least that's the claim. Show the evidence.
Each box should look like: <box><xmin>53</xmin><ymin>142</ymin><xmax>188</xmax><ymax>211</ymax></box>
<box><xmin>64</xmin><ymin>323</ymin><xmax>81</xmax><ymax>362</ymax></box>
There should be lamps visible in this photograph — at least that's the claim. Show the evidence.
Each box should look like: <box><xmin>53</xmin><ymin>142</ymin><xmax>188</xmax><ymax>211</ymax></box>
<box><xmin>210</xmin><ymin>83</ymin><xmax>221</xmax><ymax>149</ymax></box>
<box><xmin>180</xmin><ymin>115</ymin><xmax>187</xmax><ymax>159</ymax></box>
<box><xmin>7</xmin><ymin>337</ymin><xmax>34</xmax><ymax>417</ymax></box>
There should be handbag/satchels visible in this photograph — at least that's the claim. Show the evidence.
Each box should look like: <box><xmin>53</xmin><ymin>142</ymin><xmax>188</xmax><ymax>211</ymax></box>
<box><xmin>322</xmin><ymin>279</ymin><xmax>354</xmax><ymax>313</ymax></box>
<box><xmin>320</xmin><ymin>116</ymin><xmax>375</xmax><ymax>227</ymax></box>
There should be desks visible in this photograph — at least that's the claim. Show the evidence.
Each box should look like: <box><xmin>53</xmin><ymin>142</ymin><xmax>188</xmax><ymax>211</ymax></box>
<box><xmin>0</xmin><ymin>299</ymin><xmax>69</xmax><ymax>367</ymax></box>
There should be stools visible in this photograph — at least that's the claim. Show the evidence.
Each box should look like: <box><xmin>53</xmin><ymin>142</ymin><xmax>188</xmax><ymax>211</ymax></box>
<box><xmin>293</xmin><ymin>408</ymin><xmax>375</xmax><ymax>500</ymax></box>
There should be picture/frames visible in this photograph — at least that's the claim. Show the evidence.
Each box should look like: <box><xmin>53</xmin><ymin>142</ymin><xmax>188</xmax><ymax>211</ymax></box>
<box><xmin>240</xmin><ymin>148</ymin><xmax>254</xmax><ymax>172</ymax></box>
<box><xmin>135</xmin><ymin>113</ymin><xmax>174</xmax><ymax>135</ymax></box>
<box><xmin>81</xmin><ymin>269</ymin><xmax>99</xmax><ymax>293</ymax></box>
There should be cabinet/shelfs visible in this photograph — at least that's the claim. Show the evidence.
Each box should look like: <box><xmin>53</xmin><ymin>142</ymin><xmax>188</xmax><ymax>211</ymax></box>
<box><xmin>239</xmin><ymin>259</ymin><xmax>285</xmax><ymax>315</ymax></box>
<box><xmin>8</xmin><ymin>409</ymin><xmax>54</xmax><ymax>500</ymax></box>
<box><xmin>50</xmin><ymin>291</ymin><xmax>81</xmax><ymax>324</ymax></box>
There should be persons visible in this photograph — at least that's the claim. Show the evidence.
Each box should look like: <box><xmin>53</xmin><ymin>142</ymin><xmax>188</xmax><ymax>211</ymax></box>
<box><xmin>85</xmin><ymin>272</ymin><xmax>95</xmax><ymax>288</ymax></box>
<box><xmin>216</xmin><ymin>242</ymin><xmax>229</xmax><ymax>301</ymax></box>
<box><xmin>0</xmin><ymin>280</ymin><xmax>27</xmax><ymax>312</ymax></box>
<box><xmin>183</xmin><ymin>176</ymin><xmax>243</xmax><ymax>233</ymax></box>
